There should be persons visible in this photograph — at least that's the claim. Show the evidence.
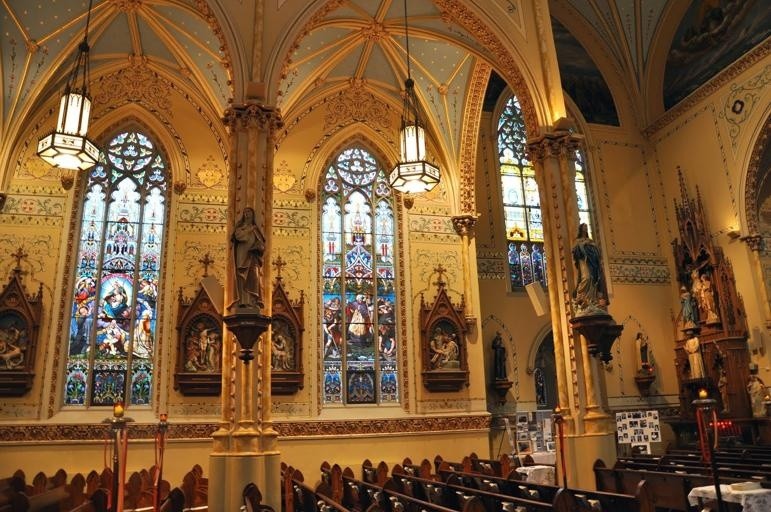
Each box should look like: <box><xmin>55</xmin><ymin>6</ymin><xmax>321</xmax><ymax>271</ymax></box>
<box><xmin>270</xmin><ymin>332</ymin><xmax>291</xmax><ymax>371</ymax></box>
<box><xmin>186</xmin><ymin>328</ymin><xmax>221</xmax><ymax>372</ymax></box>
<box><xmin>492</xmin><ymin>331</ymin><xmax>508</xmax><ymax>382</ymax></box>
<box><xmin>572</xmin><ymin>222</ymin><xmax>611</xmax><ymax>315</ymax></box>
<box><xmin>635</xmin><ymin>332</ymin><xmax>650</xmax><ymax>371</ymax></box>
<box><xmin>679</xmin><ymin>269</ymin><xmax>730</xmax><ymax>415</ymax></box>
<box><xmin>746</xmin><ymin>375</ymin><xmax>767</xmax><ymax>417</ymax></box>
<box><xmin>70</xmin><ymin>278</ymin><xmax>157</xmax><ymax>358</ymax></box>
<box><xmin>429</xmin><ymin>327</ymin><xmax>460</xmax><ymax>369</ymax></box>
<box><xmin>324</xmin><ymin>294</ymin><xmax>395</xmax><ymax>359</ymax></box>
<box><xmin>226</xmin><ymin>207</ymin><xmax>266</xmax><ymax>314</ymax></box>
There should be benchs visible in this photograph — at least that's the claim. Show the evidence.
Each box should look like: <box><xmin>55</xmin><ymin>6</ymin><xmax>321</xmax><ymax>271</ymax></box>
<box><xmin>0</xmin><ymin>440</ymin><xmax>771</xmax><ymax>512</ymax></box>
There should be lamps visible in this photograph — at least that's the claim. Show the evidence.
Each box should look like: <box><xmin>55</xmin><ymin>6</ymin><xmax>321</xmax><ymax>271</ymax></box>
<box><xmin>35</xmin><ymin>0</ymin><xmax>107</xmax><ymax>172</ymax></box>
<box><xmin>386</xmin><ymin>1</ymin><xmax>440</xmax><ymax>197</ymax></box>
